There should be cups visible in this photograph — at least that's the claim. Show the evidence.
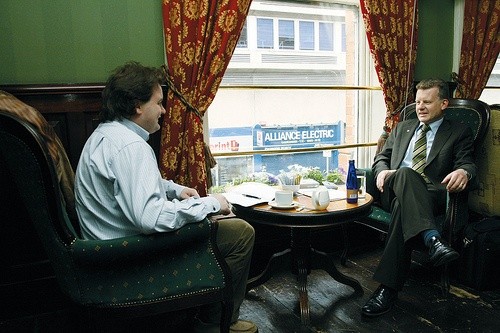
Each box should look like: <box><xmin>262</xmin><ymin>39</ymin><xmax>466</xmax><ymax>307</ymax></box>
<box><xmin>356</xmin><ymin>175</ymin><xmax>366</xmax><ymax>199</ymax></box>
<box><xmin>274</xmin><ymin>190</ymin><xmax>293</xmax><ymax>206</ymax></box>
<box><xmin>312</xmin><ymin>189</ymin><xmax>330</xmax><ymax>210</ymax></box>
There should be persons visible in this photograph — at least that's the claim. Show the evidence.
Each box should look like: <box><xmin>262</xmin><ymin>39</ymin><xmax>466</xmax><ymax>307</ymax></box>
<box><xmin>361</xmin><ymin>79</ymin><xmax>477</xmax><ymax>316</ymax></box>
<box><xmin>73</xmin><ymin>61</ymin><xmax>255</xmax><ymax>333</ymax></box>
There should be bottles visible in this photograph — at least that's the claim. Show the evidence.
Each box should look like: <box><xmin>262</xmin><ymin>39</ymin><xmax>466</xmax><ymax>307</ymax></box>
<box><xmin>346</xmin><ymin>160</ymin><xmax>358</xmax><ymax>203</ymax></box>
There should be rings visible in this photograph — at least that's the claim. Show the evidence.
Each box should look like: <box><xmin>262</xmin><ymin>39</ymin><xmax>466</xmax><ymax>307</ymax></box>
<box><xmin>461</xmin><ymin>183</ymin><xmax>464</xmax><ymax>185</ymax></box>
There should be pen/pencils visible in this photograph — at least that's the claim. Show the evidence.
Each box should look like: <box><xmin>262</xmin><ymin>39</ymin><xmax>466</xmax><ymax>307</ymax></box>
<box><xmin>242</xmin><ymin>194</ymin><xmax>261</xmax><ymax>199</ymax></box>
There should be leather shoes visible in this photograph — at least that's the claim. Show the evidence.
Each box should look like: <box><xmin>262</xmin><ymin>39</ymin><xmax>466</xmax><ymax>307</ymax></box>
<box><xmin>361</xmin><ymin>284</ymin><xmax>398</xmax><ymax>318</ymax></box>
<box><xmin>427</xmin><ymin>235</ymin><xmax>460</xmax><ymax>269</ymax></box>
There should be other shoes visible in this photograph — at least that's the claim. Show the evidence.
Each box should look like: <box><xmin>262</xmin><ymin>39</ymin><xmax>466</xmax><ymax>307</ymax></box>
<box><xmin>228</xmin><ymin>319</ymin><xmax>258</xmax><ymax>333</ymax></box>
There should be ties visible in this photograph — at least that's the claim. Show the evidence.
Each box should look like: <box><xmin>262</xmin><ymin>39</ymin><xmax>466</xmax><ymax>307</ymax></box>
<box><xmin>411</xmin><ymin>124</ymin><xmax>431</xmax><ymax>184</ymax></box>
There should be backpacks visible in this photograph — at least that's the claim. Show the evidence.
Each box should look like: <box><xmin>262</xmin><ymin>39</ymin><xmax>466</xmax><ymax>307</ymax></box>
<box><xmin>461</xmin><ymin>215</ymin><xmax>500</xmax><ymax>257</ymax></box>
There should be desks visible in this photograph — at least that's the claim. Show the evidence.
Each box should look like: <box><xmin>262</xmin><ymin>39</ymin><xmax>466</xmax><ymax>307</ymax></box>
<box><xmin>231</xmin><ymin>181</ymin><xmax>373</xmax><ymax>325</ymax></box>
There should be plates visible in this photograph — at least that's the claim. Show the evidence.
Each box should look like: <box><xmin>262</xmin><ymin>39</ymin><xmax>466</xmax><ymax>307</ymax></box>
<box><xmin>268</xmin><ymin>201</ymin><xmax>298</xmax><ymax>208</ymax></box>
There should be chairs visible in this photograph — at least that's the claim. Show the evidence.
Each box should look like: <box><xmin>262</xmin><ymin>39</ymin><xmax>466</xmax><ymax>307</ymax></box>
<box><xmin>0</xmin><ymin>86</ymin><xmax>234</xmax><ymax>333</ymax></box>
<box><xmin>350</xmin><ymin>99</ymin><xmax>490</xmax><ymax>277</ymax></box>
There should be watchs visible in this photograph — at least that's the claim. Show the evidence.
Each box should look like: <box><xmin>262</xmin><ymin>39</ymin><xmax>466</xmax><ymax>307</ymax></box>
<box><xmin>465</xmin><ymin>170</ymin><xmax>472</xmax><ymax>180</ymax></box>
